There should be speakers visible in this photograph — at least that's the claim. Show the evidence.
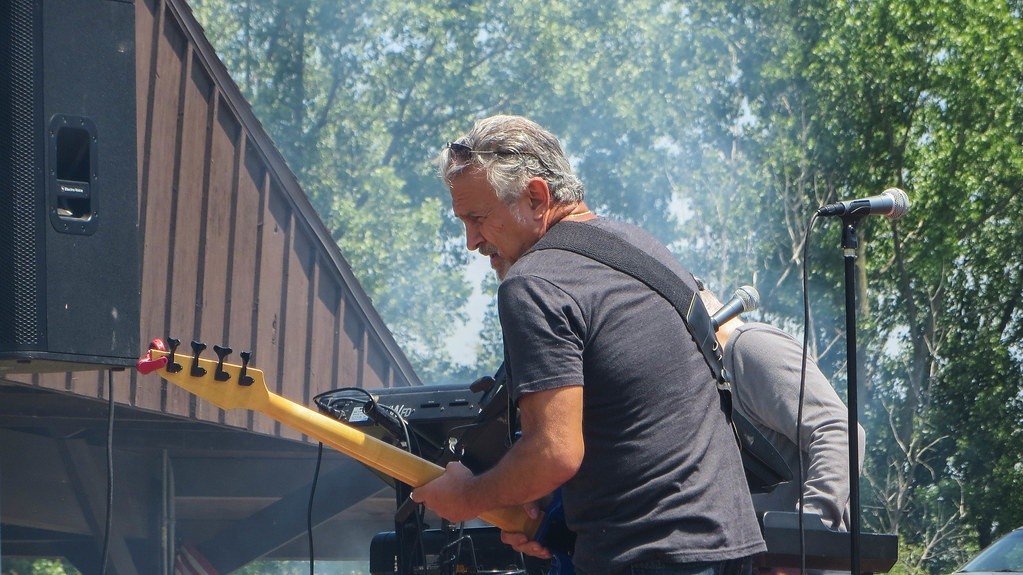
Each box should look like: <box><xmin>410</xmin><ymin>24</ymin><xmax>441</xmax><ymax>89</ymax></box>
<box><xmin>0</xmin><ymin>2</ymin><xmax>143</xmax><ymax>372</ymax></box>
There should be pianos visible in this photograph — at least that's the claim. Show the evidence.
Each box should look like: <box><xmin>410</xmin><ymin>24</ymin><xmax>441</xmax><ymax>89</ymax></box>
<box><xmin>315</xmin><ymin>376</ymin><xmax>899</xmax><ymax>575</ymax></box>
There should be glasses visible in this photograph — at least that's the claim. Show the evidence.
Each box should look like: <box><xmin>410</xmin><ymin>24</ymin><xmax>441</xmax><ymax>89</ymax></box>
<box><xmin>448</xmin><ymin>141</ymin><xmax>556</xmax><ymax>178</ymax></box>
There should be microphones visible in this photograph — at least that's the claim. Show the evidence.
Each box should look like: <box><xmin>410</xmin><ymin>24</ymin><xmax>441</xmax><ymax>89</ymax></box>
<box><xmin>709</xmin><ymin>284</ymin><xmax>759</xmax><ymax>332</ymax></box>
<box><xmin>818</xmin><ymin>187</ymin><xmax>908</xmax><ymax>220</ymax></box>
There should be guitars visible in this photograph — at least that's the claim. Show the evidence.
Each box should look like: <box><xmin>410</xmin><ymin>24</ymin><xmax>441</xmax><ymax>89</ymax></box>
<box><xmin>148</xmin><ymin>339</ymin><xmax>570</xmax><ymax>555</ymax></box>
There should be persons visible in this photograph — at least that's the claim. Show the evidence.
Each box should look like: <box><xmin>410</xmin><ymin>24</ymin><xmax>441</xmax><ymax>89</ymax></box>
<box><xmin>694</xmin><ymin>279</ymin><xmax>866</xmax><ymax>575</ymax></box>
<box><xmin>409</xmin><ymin>117</ymin><xmax>769</xmax><ymax>574</ymax></box>
